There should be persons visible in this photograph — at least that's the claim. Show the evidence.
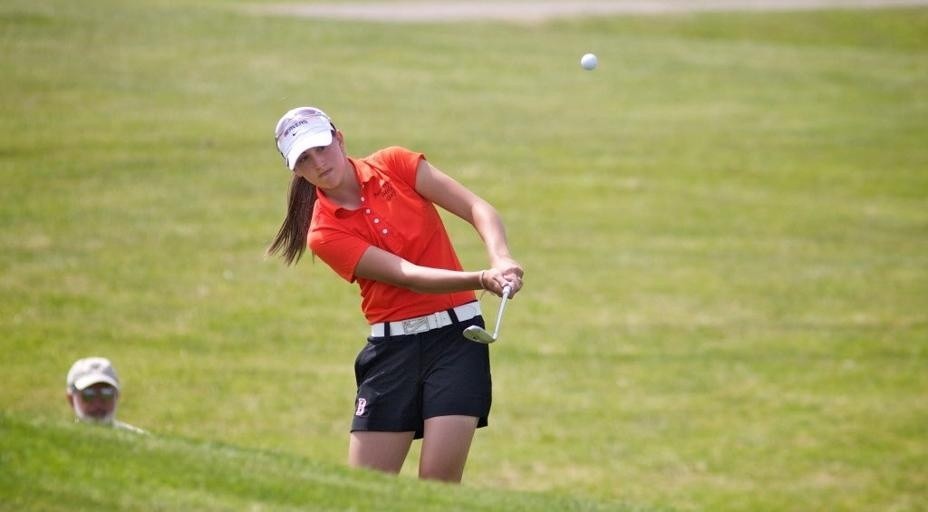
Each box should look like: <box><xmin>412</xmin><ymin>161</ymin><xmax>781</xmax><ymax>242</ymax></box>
<box><xmin>266</xmin><ymin>104</ymin><xmax>525</xmax><ymax>483</ymax></box>
<box><xmin>64</xmin><ymin>355</ymin><xmax>149</xmax><ymax>437</ymax></box>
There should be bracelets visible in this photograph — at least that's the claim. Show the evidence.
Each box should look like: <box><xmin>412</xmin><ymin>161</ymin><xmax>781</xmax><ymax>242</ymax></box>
<box><xmin>479</xmin><ymin>269</ymin><xmax>494</xmax><ymax>295</ymax></box>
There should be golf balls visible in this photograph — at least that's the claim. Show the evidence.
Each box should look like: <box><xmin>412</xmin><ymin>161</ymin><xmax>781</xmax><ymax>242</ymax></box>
<box><xmin>582</xmin><ymin>54</ymin><xmax>597</xmax><ymax>70</ymax></box>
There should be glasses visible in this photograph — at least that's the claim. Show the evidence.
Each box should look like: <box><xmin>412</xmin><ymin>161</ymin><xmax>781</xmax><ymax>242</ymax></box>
<box><xmin>81</xmin><ymin>387</ymin><xmax>117</xmax><ymax>400</ymax></box>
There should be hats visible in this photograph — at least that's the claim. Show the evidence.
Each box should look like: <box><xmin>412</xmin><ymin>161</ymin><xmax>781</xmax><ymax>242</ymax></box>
<box><xmin>275</xmin><ymin>106</ymin><xmax>335</xmax><ymax>170</ymax></box>
<box><xmin>67</xmin><ymin>357</ymin><xmax>120</xmax><ymax>393</ymax></box>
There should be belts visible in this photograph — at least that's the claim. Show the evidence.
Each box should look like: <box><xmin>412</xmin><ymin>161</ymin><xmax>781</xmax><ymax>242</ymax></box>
<box><xmin>370</xmin><ymin>301</ymin><xmax>482</xmax><ymax>337</ymax></box>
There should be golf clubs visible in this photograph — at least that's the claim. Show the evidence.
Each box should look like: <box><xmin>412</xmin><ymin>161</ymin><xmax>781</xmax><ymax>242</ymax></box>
<box><xmin>463</xmin><ymin>286</ymin><xmax>510</xmax><ymax>344</ymax></box>
